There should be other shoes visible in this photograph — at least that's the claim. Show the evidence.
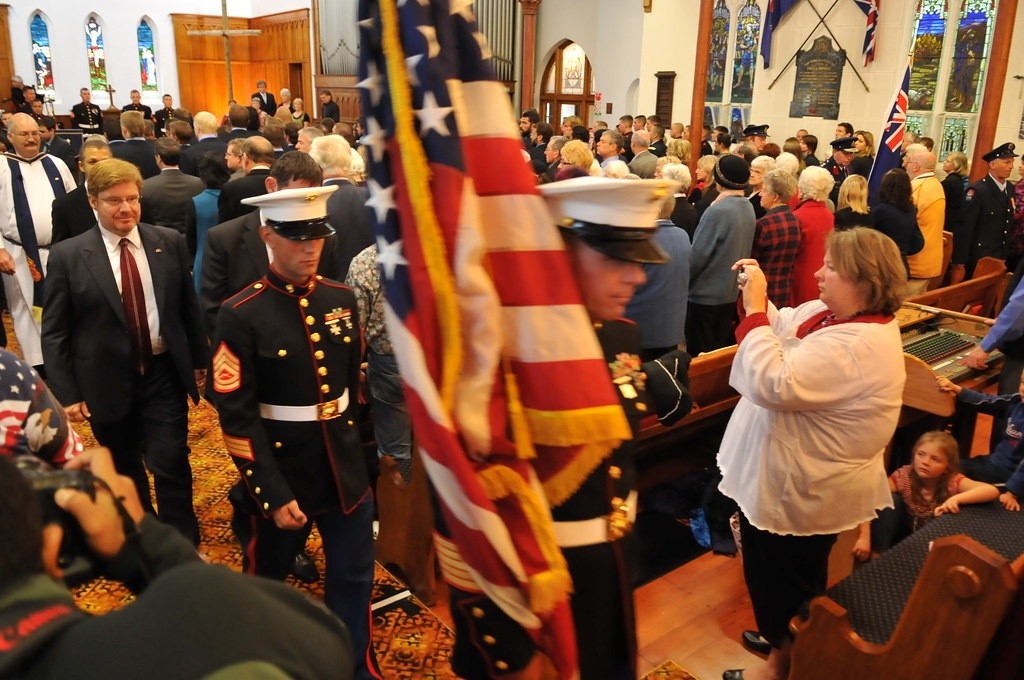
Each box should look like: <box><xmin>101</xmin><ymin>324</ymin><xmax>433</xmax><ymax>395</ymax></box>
<box><xmin>742</xmin><ymin>630</ymin><xmax>775</xmax><ymax>658</ymax></box>
<box><xmin>722</xmin><ymin>669</ymin><xmax>747</xmax><ymax>680</ymax></box>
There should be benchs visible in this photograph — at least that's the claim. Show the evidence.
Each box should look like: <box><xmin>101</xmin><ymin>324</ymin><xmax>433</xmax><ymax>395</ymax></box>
<box><xmin>788</xmin><ymin>473</ymin><xmax>1024</xmax><ymax>680</ymax></box>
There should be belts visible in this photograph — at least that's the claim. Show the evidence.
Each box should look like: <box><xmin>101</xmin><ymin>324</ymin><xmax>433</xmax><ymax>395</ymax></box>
<box><xmin>79</xmin><ymin>124</ymin><xmax>99</xmax><ymax>129</ymax></box>
<box><xmin>258</xmin><ymin>388</ymin><xmax>350</xmax><ymax>421</ymax></box>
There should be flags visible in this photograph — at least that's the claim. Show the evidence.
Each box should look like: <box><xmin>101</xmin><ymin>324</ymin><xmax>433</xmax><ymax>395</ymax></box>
<box><xmin>853</xmin><ymin>0</ymin><xmax>880</xmax><ymax>66</ymax></box>
<box><xmin>760</xmin><ymin>0</ymin><xmax>798</xmax><ymax>69</ymax></box>
<box><xmin>355</xmin><ymin>0</ymin><xmax>631</xmax><ymax>680</ymax></box>
<box><xmin>865</xmin><ymin>64</ymin><xmax>910</xmax><ymax>211</ymax></box>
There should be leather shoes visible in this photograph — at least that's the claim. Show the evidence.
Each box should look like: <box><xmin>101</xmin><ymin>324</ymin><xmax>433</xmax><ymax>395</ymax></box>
<box><xmin>290</xmin><ymin>552</ymin><xmax>320</xmax><ymax>583</ymax></box>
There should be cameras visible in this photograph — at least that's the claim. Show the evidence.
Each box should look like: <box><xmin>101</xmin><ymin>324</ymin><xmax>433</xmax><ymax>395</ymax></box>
<box><xmin>737</xmin><ymin>267</ymin><xmax>747</xmax><ymax>287</ymax></box>
<box><xmin>14</xmin><ymin>456</ymin><xmax>96</xmax><ymax>548</ymax></box>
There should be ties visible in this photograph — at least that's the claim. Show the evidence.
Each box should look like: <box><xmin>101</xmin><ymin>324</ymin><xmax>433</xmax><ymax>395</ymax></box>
<box><xmin>118</xmin><ymin>238</ymin><xmax>153</xmax><ymax>375</ymax></box>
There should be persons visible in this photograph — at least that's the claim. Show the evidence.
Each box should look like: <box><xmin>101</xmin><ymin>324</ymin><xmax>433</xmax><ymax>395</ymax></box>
<box><xmin>0</xmin><ymin>75</ymin><xmax>1024</xmax><ymax>680</ymax></box>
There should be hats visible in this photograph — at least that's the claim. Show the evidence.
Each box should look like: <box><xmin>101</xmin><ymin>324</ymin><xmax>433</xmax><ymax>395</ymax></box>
<box><xmin>830</xmin><ymin>137</ymin><xmax>859</xmax><ymax>153</ymax></box>
<box><xmin>981</xmin><ymin>142</ymin><xmax>1020</xmax><ymax>164</ymax></box>
<box><xmin>743</xmin><ymin>124</ymin><xmax>770</xmax><ymax>137</ymax></box>
<box><xmin>535</xmin><ymin>176</ymin><xmax>682</xmax><ymax>265</ymax></box>
<box><xmin>714</xmin><ymin>155</ymin><xmax>750</xmax><ymax>190</ymax></box>
<box><xmin>240</xmin><ymin>185</ymin><xmax>339</xmax><ymax>241</ymax></box>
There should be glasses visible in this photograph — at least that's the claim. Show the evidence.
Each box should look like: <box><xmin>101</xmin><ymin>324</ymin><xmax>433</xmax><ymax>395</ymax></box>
<box><xmin>90</xmin><ymin>192</ymin><xmax>144</xmax><ymax>206</ymax></box>
<box><xmin>8</xmin><ymin>130</ymin><xmax>40</xmax><ymax>136</ymax></box>
